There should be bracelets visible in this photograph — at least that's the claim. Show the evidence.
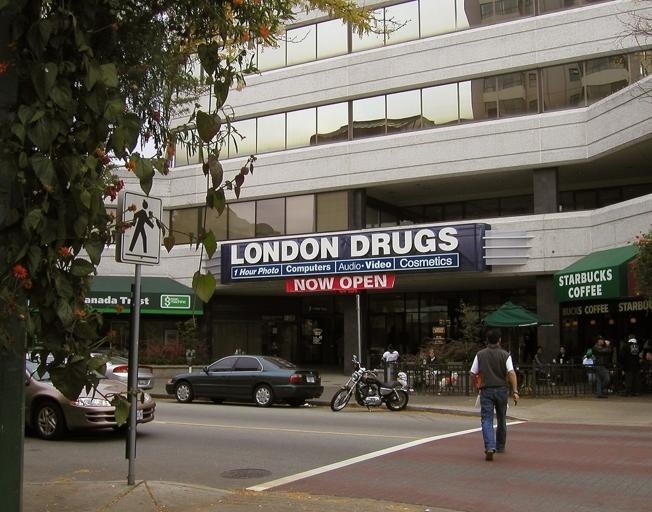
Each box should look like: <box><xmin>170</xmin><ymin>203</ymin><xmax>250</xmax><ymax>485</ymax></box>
<box><xmin>513</xmin><ymin>392</ymin><xmax>519</xmax><ymax>396</ymax></box>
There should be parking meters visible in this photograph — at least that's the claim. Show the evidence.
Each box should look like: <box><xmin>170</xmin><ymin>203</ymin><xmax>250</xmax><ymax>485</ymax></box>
<box><xmin>185</xmin><ymin>348</ymin><xmax>197</xmax><ymax>374</ymax></box>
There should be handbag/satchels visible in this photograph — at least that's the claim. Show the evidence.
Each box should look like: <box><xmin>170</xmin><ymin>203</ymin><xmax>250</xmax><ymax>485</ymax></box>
<box><xmin>474</xmin><ymin>394</ymin><xmax>481</xmax><ymax>409</ymax></box>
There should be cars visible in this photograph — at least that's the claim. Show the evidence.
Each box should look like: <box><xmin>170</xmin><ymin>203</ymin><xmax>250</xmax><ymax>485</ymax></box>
<box><xmin>165</xmin><ymin>354</ymin><xmax>324</xmax><ymax>408</ymax></box>
<box><xmin>79</xmin><ymin>351</ymin><xmax>155</xmax><ymax>389</ymax></box>
<box><xmin>25</xmin><ymin>350</ymin><xmax>156</xmax><ymax>439</ymax></box>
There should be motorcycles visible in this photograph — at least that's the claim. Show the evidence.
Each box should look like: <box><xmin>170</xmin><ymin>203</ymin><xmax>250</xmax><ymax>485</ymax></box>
<box><xmin>330</xmin><ymin>354</ymin><xmax>414</xmax><ymax>412</ymax></box>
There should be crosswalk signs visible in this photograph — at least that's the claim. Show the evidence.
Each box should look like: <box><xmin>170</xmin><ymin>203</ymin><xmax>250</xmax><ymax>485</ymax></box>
<box><xmin>118</xmin><ymin>190</ymin><xmax>163</xmax><ymax>266</ymax></box>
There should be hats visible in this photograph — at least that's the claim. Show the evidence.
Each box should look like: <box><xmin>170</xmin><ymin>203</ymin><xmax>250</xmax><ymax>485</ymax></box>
<box><xmin>487</xmin><ymin>330</ymin><xmax>500</xmax><ymax>345</ymax></box>
<box><xmin>387</xmin><ymin>343</ymin><xmax>396</xmax><ymax>353</ymax></box>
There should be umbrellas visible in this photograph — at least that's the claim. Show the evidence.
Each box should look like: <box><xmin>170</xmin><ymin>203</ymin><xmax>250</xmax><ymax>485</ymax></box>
<box><xmin>482</xmin><ymin>301</ymin><xmax>555</xmax><ymax>355</ymax></box>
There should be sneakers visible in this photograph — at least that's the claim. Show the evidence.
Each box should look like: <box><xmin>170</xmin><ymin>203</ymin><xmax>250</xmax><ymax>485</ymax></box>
<box><xmin>485</xmin><ymin>448</ymin><xmax>505</xmax><ymax>461</ymax></box>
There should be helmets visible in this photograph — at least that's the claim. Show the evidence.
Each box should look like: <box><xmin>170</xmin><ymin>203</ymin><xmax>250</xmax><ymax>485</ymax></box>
<box><xmin>397</xmin><ymin>372</ymin><xmax>408</xmax><ymax>381</ymax></box>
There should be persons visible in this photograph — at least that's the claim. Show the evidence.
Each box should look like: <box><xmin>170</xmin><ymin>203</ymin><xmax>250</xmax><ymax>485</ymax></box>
<box><xmin>382</xmin><ymin>345</ymin><xmax>399</xmax><ymax>364</ymax></box>
<box><xmin>471</xmin><ymin>330</ymin><xmax>520</xmax><ymax>460</ymax></box>
<box><xmin>424</xmin><ymin>348</ymin><xmax>440</xmax><ymax>387</ymax></box>
<box><xmin>534</xmin><ymin>335</ymin><xmax>651</xmax><ymax>399</ymax></box>
